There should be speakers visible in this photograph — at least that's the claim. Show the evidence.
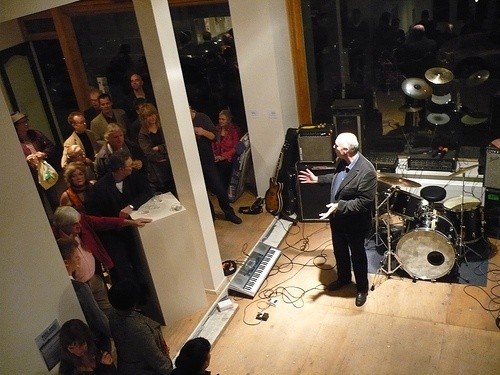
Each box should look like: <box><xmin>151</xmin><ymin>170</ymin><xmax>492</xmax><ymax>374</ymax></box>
<box><xmin>295</xmin><ymin>129</ymin><xmax>335</xmax><ymax>162</ymax></box>
<box><xmin>296</xmin><ymin>159</ymin><xmax>335</xmax><ymax>221</ymax></box>
<box><xmin>485</xmin><ymin>147</ymin><xmax>500</xmax><ymax>193</ymax></box>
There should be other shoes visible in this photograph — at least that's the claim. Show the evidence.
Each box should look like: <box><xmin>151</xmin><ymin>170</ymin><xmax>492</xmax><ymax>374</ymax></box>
<box><xmin>225</xmin><ymin>213</ymin><xmax>243</xmax><ymax>224</ymax></box>
<box><xmin>212</xmin><ymin>213</ymin><xmax>215</xmax><ymax>222</ymax></box>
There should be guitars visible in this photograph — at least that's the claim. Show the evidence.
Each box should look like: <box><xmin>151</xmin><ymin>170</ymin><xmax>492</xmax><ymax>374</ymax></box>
<box><xmin>263</xmin><ymin>142</ymin><xmax>291</xmax><ymax>215</ymax></box>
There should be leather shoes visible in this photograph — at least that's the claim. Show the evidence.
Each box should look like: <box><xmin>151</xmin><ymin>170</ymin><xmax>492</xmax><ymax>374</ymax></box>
<box><xmin>355</xmin><ymin>291</ymin><xmax>367</xmax><ymax>306</ymax></box>
<box><xmin>328</xmin><ymin>280</ymin><xmax>344</xmax><ymax>291</ymax></box>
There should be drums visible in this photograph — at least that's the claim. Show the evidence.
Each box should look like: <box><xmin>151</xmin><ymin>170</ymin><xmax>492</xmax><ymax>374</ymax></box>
<box><xmin>396</xmin><ymin>213</ymin><xmax>458</xmax><ymax>282</ymax></box>
<box><xmin>385</xmin><ymin>187</ymin><xmax>421</xmax><ymax>221</ymax></box>
<box><xmin>376</xmin><ymin>215</ymin><xmax>407</xmax><ymax>250</ymax></box>
<box><xmin>443</xmin><ymin>195</ymin><xmax>484</xmax><ymax>247</ymax></box>
<box><xmin>428</xmin><ymin>90</ymin><xmax>452</xmax><ymax>113</ymax></box>
<box><xmin>461</xmin><ymin>115</ymin><xmax>489</xmax><ymax>146</ymax></box>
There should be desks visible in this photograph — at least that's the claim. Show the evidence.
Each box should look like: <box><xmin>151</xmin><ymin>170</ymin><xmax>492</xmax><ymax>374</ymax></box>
<box><xmin>127</xmin><ymin>191</ymin><xmax>209</xmax><ymax>326</ymax></box>
<box><xmin>395</xmin><ymin>159</ymin><xmax>485</xmax><ymax>209</ymax></box>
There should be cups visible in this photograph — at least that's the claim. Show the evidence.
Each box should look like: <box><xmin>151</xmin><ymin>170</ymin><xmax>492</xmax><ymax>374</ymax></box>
<box><xmin>154</xmin><ymin>192</ymin><xmax>163</xmax><ymax>202</ymax></box>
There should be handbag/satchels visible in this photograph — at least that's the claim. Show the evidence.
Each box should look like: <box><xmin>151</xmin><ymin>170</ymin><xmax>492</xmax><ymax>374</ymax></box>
<box><xmin>37</xmin><ymin>159</ymin><xmax>59</xmax><ymax>191</ymax></box>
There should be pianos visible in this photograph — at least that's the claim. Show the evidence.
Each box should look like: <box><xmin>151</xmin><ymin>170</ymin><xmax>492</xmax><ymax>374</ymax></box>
<box><xmin>228</xmin><ymin>242</ymin><xmax>282</xmax><ymax>300</ymax></box>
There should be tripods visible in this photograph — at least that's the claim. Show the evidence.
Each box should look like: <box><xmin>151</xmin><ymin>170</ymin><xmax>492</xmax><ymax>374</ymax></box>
<box><xmin>363</xmin><ymin>171</ymin><xmax>484</xmax><ymax>291</ymax></box>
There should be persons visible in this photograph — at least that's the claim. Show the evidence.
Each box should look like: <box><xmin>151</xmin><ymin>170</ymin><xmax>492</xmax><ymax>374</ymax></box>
<box><xmin>296</xmin><ymin>132</ymin><xmax>378</xmax><ymax>306</ymax></box>
<box><xmin>52</xmin><ymin>206</ymin><xmax>152</xmax><ymax>325</ymax></box>
<box><xmin>168</xmin><ymin>338</ymin><xmax>213</xmax><ymax>375</ymax></box>
<box><xmin>57</xmin><ymin>319</ymin><xmax>116</xmax><ymax>375</ymax></box>
<box><xmin>55</xmin><ymin>237</ymin><xmax>110</xmax><ymax>345</ymax></box>
<box><xmin>347</xmin><ymin>5</ymin><xmax>464</xmax><ymax>109</ymax></box>
<box><xmin>2</xmin><ymin>68</ymin><xmax>242</xmax><ymax>318</ymax></box>
<box><xmin>106</xmin><ymin>282</ymin><xmax>175</xmax><ymax>375</ymax></box>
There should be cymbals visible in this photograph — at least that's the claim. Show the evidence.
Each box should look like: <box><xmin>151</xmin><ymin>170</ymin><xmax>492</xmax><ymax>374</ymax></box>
<box><xmin>401</xmin><ymin>79</ymin><xmax>432</xmax><ymax>98</ymax></box>
<box><xmin>425</xmin><ymin>67</ymin><xmax>454</xmax><ymax>84</ymax></box>
<box><xmin>448</xmin><ymin>164</ymin><xmax>480</xmax><ymax>178</ymax></box>
<box><xmin>377</xmin><ymin>176</ymin><xmax>422</xmax><ymax>188</ymax></box>
<box><xmin>399</xmin><ymin>103</ymin><xmax>423</xmax><ymax>112</ymax></box>
<box><xmin>466</xmin><ymin>71</ymin><xmax>489</xmax><ymax>85</ymax></box>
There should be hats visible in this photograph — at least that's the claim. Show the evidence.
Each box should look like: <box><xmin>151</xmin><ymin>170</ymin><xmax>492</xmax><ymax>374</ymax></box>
<box><xmin>174</xmin><ymin>337</ymin><xmax>211</xmax><ymax>371</ymax></box>
<box><xmin>10</xmin><ymin>111</ymin><xmax>28</xmax><ymax>125</ymax></box>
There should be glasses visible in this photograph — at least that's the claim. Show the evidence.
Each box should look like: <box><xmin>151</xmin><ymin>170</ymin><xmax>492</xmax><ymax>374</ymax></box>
<box><xmin>19</xmin><ymin>119</ymin><xmax>29</xmax><ymax>125</ymax></box>
<box><xmin>74</xmin><ymin>119</ymin><xmax>87</xmax><ymax>126</ymax></box>
